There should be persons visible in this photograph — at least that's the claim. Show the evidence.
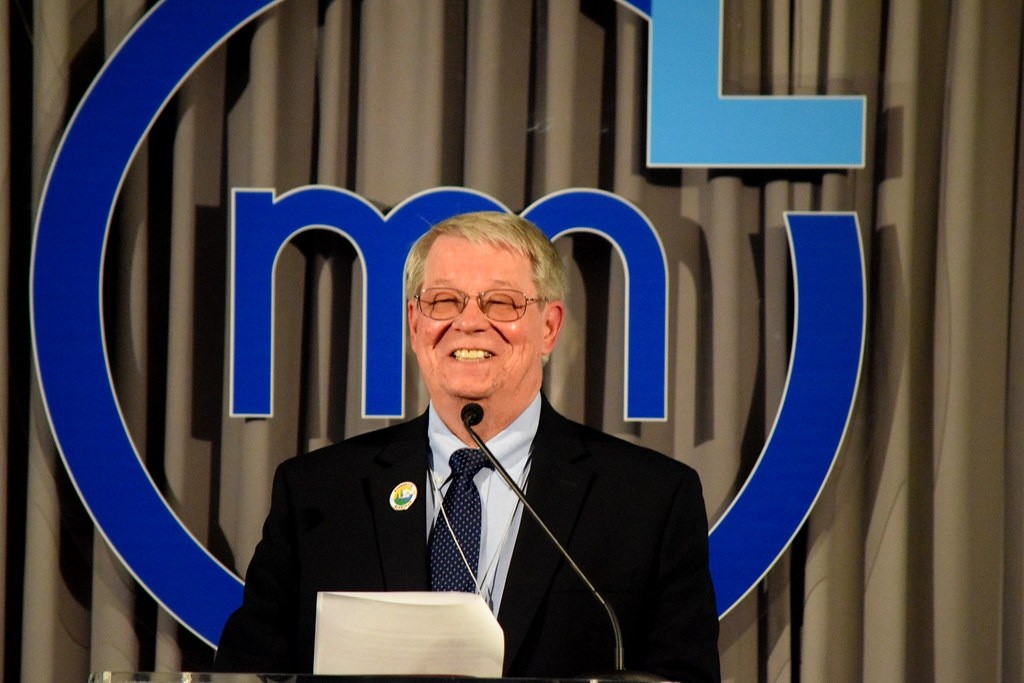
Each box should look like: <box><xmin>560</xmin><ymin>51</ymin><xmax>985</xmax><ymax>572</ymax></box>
<box><xmin>213</xmin><ymin>212</ymin><xmax>721</xmax><ymax>683</ymax></box>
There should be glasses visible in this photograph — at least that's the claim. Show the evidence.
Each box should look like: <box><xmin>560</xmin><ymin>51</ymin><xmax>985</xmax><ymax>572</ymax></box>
<box><xmin>413</xmin><ymin>288</ymin><xmax>541</xmax><ymax>322</ymax></box>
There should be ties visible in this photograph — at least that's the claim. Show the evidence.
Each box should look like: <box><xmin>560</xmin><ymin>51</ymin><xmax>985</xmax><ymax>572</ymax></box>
<box><xmin>426</xmin><ymin>448</ymin><xmax>495</xmax><ymax>592</ymax></box>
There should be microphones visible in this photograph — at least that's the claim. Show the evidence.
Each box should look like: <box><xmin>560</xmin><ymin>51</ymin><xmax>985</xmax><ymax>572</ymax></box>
<box><xmin>460</xmin><ymin>403</ymin><xmax>669</xmax><ymax>683</ymax></box>
